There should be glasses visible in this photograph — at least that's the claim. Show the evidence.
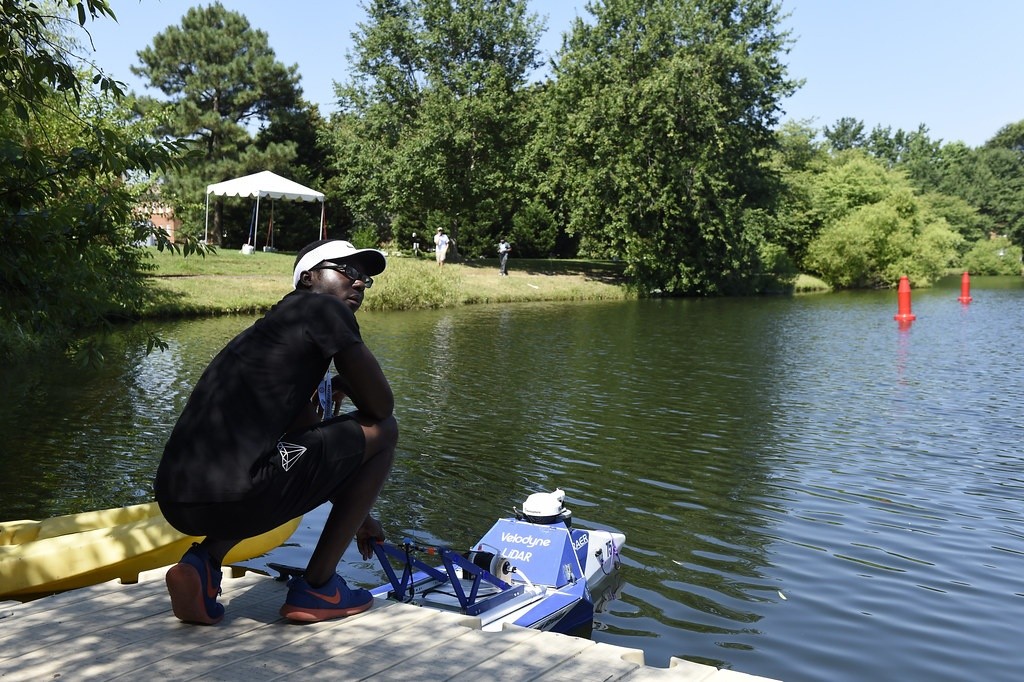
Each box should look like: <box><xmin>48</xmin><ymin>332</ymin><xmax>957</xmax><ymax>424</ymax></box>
<box><xmin>310</xmin><ymin>264</ymin><xmax>374</xmax><ymax>289</ymax></box>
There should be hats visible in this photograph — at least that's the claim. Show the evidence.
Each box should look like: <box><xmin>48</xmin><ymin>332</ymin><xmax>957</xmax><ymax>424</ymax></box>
<box><xmin>412</xmin><ymin>232</ymin><xmax>418</xmax><ymax>237</ymax></box>
<box><xmin>293</xmin><ymin>241</ymin><xmax>387</xmax><ymax>290</ymax></box>
<box><xmin>438</xmin><ymin>227</ymin><xmax>444</xmax><ymax>230</ymax></box>
<box><xmin>499</xmin><ymin>236</ymin><xmax>505</xmax><ymax>239</ymax></box>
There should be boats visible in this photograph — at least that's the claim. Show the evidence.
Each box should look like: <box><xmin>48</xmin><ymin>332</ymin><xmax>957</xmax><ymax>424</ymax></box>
<box><xmin>0</xmin><ymin>500</ymin><xmax>303</xmax><ymax>601</ymax></box>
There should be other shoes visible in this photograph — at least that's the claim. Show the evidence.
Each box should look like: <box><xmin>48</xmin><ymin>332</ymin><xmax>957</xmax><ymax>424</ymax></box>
<box><xmin>499</xmin><ymin>273</ymin><xmax>503</xmax><ymax>276</ymax></box>
<box><xmin>503</xmin><ymin>273</ymin><xmax>506</xmax><ymax>276</ymax></box>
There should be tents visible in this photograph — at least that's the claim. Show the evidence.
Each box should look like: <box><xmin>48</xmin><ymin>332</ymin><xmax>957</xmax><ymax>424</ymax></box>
<box><xmin>204</xmin><ymin>169</ymin><xmax>325</xmax><ymax>253</ymax></box>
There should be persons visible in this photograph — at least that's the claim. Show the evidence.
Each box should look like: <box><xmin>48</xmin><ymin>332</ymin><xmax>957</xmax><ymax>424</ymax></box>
<box><xmin>412</xmin><ymin>232</ymin><xmax>421</xmax><ymax>252</ymax></box>
<box><xmin>497</xmin><ymin>237</ymin><xmax>512</xmax><ymax>277</ymax></box>
<box><xmin>434</xmin><ymin>227</ymin><xmax>450</xmax><ymax>267</ymax></box>
<box><xmin>156</xmin><ymin>239</ymin><xmax>400</xmax><ymax>626</ymax></box>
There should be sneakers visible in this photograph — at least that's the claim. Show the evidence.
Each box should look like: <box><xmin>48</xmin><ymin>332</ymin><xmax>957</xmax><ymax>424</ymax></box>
<box><xmin>166</xmin><ymin>544</ymin><xmax>225</xmax><ymax>626</ymax></box>
<box><xmin>280</xmin><ymin>570</ymin><xmax>373</xmax><ymax>621</ymax></box>
